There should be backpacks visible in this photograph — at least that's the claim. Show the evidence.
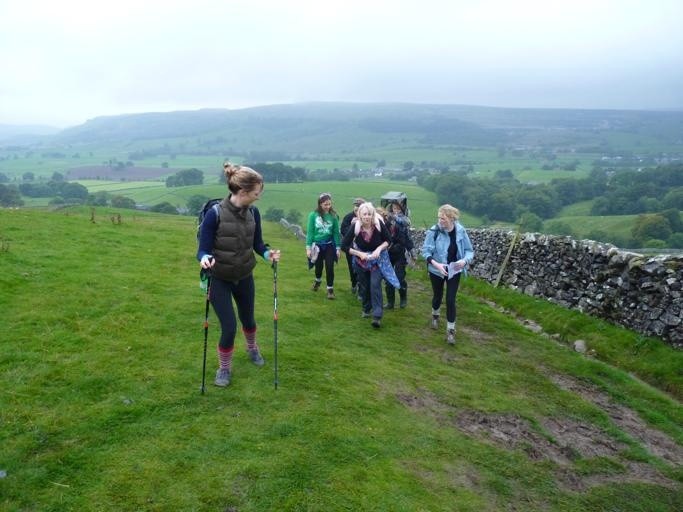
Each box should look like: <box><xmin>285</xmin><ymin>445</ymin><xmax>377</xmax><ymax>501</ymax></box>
<box><xmin>196</xmin><ymin>198</ymin><xmax>254</xmax><ymax>240</ymax></box>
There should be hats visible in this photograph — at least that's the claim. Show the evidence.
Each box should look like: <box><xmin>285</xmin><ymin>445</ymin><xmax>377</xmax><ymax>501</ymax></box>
<box><xmin>352</xmin><ymin>198</ymin><xmax>365</xmax><ymax>204</ymax></box>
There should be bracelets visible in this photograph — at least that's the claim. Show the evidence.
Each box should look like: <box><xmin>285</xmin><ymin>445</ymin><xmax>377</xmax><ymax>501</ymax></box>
<box><xmin>263</xmin><ymin>250</ymin><xmax>268</xmax><ymax>260</ymax></box>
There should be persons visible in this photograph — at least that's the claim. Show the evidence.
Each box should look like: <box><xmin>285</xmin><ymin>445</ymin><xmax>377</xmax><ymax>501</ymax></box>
<box><xmin>421</xmin><ymin>204</ymin><xmax>474</xmax><ymax>344</ymax></box>
<box><xmin>196</xmin><ymin>163</ymin><xmax>280</xmax><ymax>386</ymax></box>
<box><xmin>341</xmin><ymin>198</ymin><xmax>417</xmax><ymax>328</ymax></box>
<box><xmin>305</xmin><ymin>193</ymin><xmax>342</xmax><ymax>299</ymax></box>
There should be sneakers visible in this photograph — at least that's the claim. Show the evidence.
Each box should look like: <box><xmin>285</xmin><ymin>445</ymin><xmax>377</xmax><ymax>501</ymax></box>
<box><xmin>311</xmin><ymin>280</ymin><xmax>321</xmax><ymax>291</ymax></box>
<box><xmin>214</xmin><ymin>367</ymin><xmax>231</xmax><ymax>387</ymax></box>
<box><xmin>249</xmin><ymin>345</ymin><xmax>264</xmax><ymax>366</ymax></box>
<box><xmin>431</xmin><ymin>315</ymin><xmax>438</xmax><ymax>330</ymax></box>
<box><xmin>446</xmin><ymin>329</ymin><xmax>456</xmax><ymax>344</ymax></box>
<box><xmin>351</xmin><ymin>285</ymin><xmax>406</xmax><ymax>328</ymax></box>
<box><xmin>327</xmin><ymin>289</ymin><xmax>335</xmax><ymax>299</ymax></box>
